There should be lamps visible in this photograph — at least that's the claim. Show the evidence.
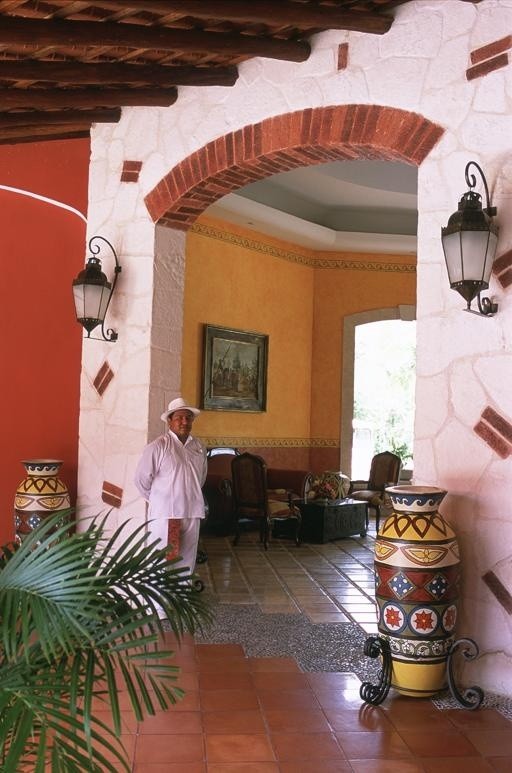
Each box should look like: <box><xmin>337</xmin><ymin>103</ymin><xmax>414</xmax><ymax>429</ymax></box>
<box><xmin>440</xmin><ymin>161</ymin><xmax>499</xmax><ymax>318</ymax></box>
<box><xmin>73</xmin><ymin>236</ymin><xmax>121</xmax><ymax>343</ymax></box>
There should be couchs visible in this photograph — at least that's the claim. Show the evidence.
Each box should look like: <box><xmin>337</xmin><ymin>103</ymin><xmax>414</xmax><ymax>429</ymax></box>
<box><xmin>206</xmin><ymin>468</ymin><xmax>307</xmax><ymax>538</ymax></box>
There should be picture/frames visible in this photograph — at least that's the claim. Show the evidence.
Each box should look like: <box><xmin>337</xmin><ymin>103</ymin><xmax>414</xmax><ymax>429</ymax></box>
<box><xmin>201</xmin><ymin>323</ymin><xmax>269</xmax><ymax>413</ymax></box>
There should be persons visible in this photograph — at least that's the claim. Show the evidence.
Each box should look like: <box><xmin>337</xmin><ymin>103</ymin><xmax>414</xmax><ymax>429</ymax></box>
<box><xmin>134</xmin><ymin>398</ymin><xmax>209</xmax><ymax>631</ymax></box>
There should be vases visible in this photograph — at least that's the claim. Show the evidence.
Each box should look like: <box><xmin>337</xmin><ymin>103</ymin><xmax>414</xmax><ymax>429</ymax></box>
<box><xmin>377</xmin><ymin>486</ymin><xmax>459</xmax><ymax>697</ymax></box>
<box><xmin>14</xmin><ymin>458</ymin><xmax>71</xmax><ymax>609</ymax></box>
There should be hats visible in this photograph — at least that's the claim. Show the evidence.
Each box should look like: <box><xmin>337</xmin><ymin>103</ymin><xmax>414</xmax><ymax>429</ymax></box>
<box><xmin>160</xmin><ymin>397</ymin><xmax>200</xmax><ymax>424</ymax></box>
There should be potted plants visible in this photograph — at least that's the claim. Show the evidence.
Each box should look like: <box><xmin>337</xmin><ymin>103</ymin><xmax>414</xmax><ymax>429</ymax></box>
<box><xmin>305</xmin><ymin>471</ymin><xmax>345</xmax><ymax>502</ymax></box>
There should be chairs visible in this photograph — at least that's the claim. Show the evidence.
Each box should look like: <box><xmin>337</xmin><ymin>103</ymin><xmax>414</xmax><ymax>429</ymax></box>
<box><xmin>349</xmin><ymin>450</ymin><xmax>402</xmax><ymax>535</ymax></box>
<box><xmin>229</xmin><ymin>451</ymin><xmax>302</xmax><ymax>551</ymax></box>
